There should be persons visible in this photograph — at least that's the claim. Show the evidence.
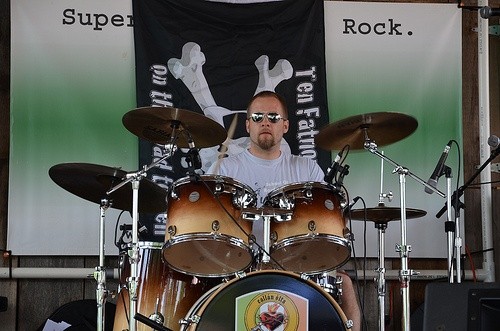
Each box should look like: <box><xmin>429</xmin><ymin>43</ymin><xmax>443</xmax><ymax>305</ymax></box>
<box><xmin>206</xmin><ymin>91</ymin><xmax>361</xmax><ymax>331</ymax></box>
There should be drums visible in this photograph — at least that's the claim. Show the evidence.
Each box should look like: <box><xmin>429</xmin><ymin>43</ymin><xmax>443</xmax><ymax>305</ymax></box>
<box><xmin>179</xmin><ymin>267</ymin><xmax>354</xmax><ymax>331</ymax></box>
<box><xmin>161</xmin><ymin>174</ymin><xmax>257</xmax><ymax>277</ymax></box>
<box><xmin>112</xmin><ymin>242</ymin><xmax>252</xmax><ymax>331</ymax></box>
<box><xmin>260</xmin><ymin>180</ymin><xmax>352</xmax><ymax>274</ymax></box>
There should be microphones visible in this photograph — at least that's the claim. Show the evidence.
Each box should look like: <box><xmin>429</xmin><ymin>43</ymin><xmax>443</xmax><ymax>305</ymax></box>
<box><xmin>480</xmin><ymin>6</ymin><xmax>500</xmax><ymax>19</ymax></box>
<box><xmin>343</xmin><ymin>196</ymin><xmax>360</xmax><ymax>215</ymax></box>
<box><xmin>425</xmin><ymin>140</ymin><xmax>453</xmax><ymax>195</ymax></box>
<box><xmin>127</xmin><ymin>209</ymin><xmax>159</xmax><ymax>242</ymax></box>
<box><xmin>487</xmin><ymin>135</ymin><xmax>500</xmax><ymax>147</ymax></box>
<box><xmin>324</xmin><ymin>150</ymin><xmax>343</xmax><ymax>184</ymax></box>
<box><xmin>187</xmin><ymin>137</ymin><xmax>203</xmax><ymax>169</ymax></box>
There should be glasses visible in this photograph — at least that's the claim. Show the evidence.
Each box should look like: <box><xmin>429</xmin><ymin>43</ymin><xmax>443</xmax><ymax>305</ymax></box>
<box><xmin>247</xmin><ymin>113</ymin><xmax>286</xmax><ymax>123</ymax></box>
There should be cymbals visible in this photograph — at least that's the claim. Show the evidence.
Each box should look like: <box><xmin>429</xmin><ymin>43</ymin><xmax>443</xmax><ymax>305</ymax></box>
<box><xmin>48</xmin><ymin>163</ymin><xmax>168</xmax><ymax>212</ymax></box>
<box><xmin>122</xmin><ymin>106</ymin><xmax>227</xmax><ymax>148</ymax></box>
<box><xmin>346</xmin><ymin>206</ymin><xmax>427</xmax><ymax>221</ymax></box>
<box><xmin>314</xmin><ymin>111</ymin><xmax>419</xmax><ymax>149</ymax></box>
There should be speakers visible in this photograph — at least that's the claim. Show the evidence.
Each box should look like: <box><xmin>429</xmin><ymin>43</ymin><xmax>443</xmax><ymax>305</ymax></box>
<box><xmin>423</xmin><ymin>281</ymin><xmax>500</xmax><ymax>331</ymax></box>
